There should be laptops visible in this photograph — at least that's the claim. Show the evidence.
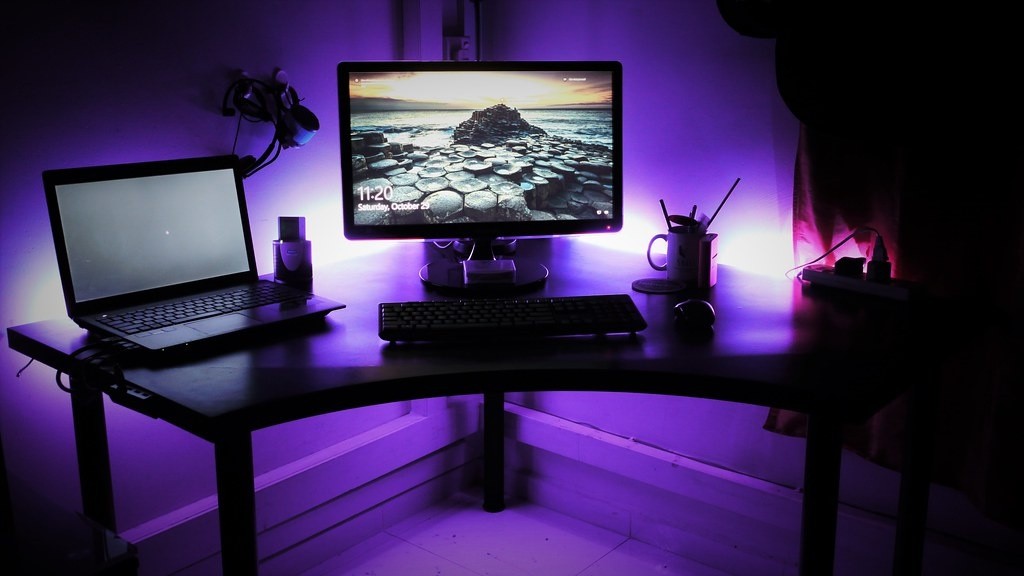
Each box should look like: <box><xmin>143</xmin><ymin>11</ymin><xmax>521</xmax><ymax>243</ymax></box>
<box><xmin>41</xmin><ymin>155</ymin><xmax>346</xmax><ymax>355</ymax></box>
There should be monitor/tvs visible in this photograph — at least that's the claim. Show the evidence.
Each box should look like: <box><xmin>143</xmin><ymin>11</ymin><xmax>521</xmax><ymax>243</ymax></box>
<box><xmin>337</xmin><ymin>59</ymin><xmax>623</xmax><ymax>243</ymax></box>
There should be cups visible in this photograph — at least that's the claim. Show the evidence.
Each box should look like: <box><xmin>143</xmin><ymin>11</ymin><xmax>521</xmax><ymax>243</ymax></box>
<box><xmin>647</xmin><ymin>225</ymin><xmax>707</xmax><ymax>283</ymax></box>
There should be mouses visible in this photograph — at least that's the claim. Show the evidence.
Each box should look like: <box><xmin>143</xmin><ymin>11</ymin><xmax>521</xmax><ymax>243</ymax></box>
<box><xmin>674</xmin><ymin>298</ymin><xmax>716</xmax><ymax>325</ymax></box>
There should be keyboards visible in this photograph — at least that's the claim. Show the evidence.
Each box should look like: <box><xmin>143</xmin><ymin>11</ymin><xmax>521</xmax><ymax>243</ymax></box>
<box><xmin>378</xmin><ymin>292</ymin><xmax>647</xmax><ymax>347</ymax></box>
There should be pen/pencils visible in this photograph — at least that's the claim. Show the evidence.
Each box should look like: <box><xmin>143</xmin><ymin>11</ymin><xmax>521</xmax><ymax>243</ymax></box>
<box><xmin>659</xmin><ymin>199</ymin><xmax>672</xmax><ymax>232</ymax></box>
<box><xmin>689</xmin><ymin>204</ymin><xmax>697</xmax><ymax>220</ymax></box>
<box><xmin>704</xmin><ymin>177</ymin><xmax>740</xmax><ymax>233</ymax></box>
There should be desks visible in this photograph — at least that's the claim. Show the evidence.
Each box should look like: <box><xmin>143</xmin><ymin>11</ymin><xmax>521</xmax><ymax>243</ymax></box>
<box><xmin>7</xmin><ymin>230</ymin><xmax>1024</xmax><ymax>576</ymax></box>
